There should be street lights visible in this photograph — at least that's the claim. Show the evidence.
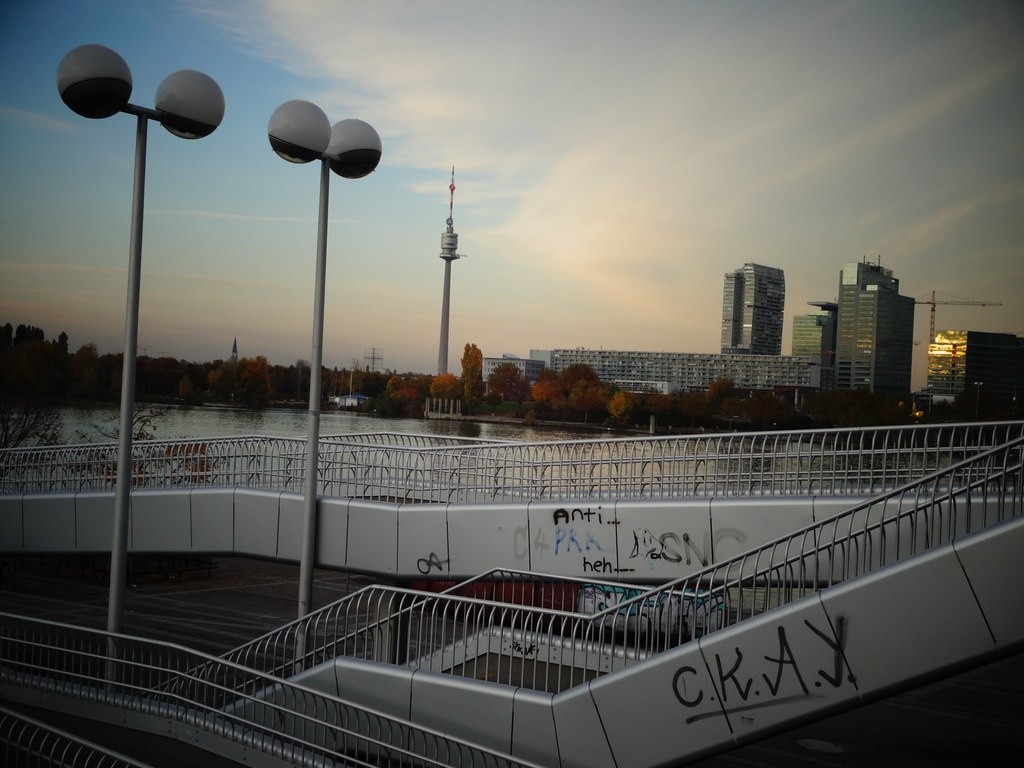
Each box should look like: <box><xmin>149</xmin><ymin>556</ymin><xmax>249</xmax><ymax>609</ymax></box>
<box><xmin>60</xmin><ymin>41</ymin><xmax>225</xmax><ymax>690</ymax></box>
<box><xmin>267</xmin><ymin>101</ymin><xmax>385</xmax><ymax>675</ymax></box>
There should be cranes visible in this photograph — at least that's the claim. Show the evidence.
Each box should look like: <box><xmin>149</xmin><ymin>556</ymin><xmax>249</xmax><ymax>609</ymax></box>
<box><xmin>915</xmin><ymin>287</ymin><xmax>1004</xmax><ymax>341</ymax></box>
